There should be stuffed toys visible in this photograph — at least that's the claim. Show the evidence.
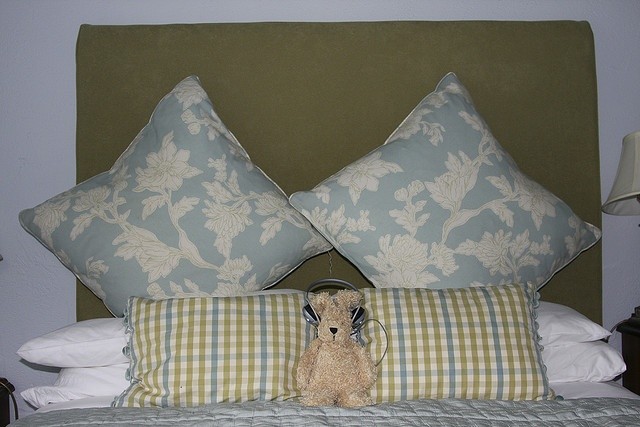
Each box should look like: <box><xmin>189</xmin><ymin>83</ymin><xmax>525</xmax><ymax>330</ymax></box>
<box><xmin>296</xmin><ymin>289</ymin><xmax>378</xmax><ymax>410</ymax></box>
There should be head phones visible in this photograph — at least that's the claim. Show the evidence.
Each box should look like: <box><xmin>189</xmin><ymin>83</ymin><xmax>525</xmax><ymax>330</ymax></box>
<box><xmin>303</xmin><ymin>278</ymin><xmax>366</xmax><ymax>335</ymax></box>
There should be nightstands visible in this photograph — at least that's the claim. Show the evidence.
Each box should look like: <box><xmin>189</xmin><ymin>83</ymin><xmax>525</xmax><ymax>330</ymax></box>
<box><xmin>614</xmin><ymin>312</ymin><xmax>640</xmax><ymax>391</ymax></box>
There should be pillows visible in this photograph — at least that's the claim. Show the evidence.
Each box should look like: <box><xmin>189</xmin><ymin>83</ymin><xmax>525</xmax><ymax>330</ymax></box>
<box><xmin>21</xmin><ymin>365</ymin><xmax>128</xmax><ymax>407</ymax></box>
<box><xmin>548</xmin><ymin>341</ymin><xmax>627</xmax><ymax>384</ymax></box>
<box><xmin>16</xmin><ymin>318</ymin><xmax>129</xmax><ymax>368</ymax></box>
<box><xmin>288</xmin><ymin>72</ymin><xmax>602</xmax><ymax>295</ymax></box>
<box><xmin>18</xmin><ymin>75</ymin><xmax>332</xmax><ymax>325</ymax></box>
<box><xmin>111</xmin><ymin>284</ymin><xmax>554</xmax><ymax>406</ymax></box>
<box><xmin>537</xmin><ymin>300</ymin><xmax>611</xmax><ymax>347</ymax></box>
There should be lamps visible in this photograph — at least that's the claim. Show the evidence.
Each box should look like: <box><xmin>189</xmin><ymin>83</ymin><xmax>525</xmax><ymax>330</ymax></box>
<box><xmin>605</xmin><ymin>127</ymin><xmax>640</xmax><ymax>325</ymax></box>
<box><xmin>601</xmin><ymin>135</ymin><xmax>639</xmax><ymax>217</ymax></box>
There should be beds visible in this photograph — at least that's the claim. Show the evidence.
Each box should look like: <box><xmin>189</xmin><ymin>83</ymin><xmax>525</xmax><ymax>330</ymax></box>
<box><xmin>0</xmin><ymin>326</ymin><xmax>640</xmax><ymax>426</ymax></box>
